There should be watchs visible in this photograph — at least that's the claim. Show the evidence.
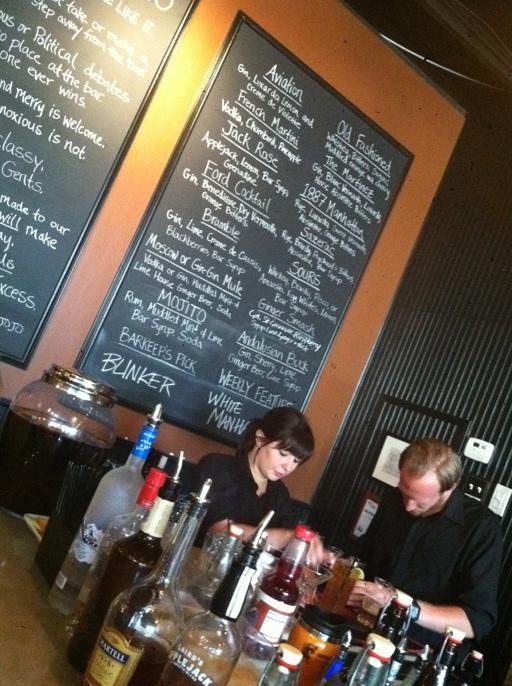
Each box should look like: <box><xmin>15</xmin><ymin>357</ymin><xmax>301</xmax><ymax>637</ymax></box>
<box><xmin>404</xmin><ymin>598</ymin><xmax>420</xmax><ymax>622</ymax></box>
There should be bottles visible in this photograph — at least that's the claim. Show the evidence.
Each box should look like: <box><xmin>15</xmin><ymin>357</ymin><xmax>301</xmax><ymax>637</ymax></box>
<box><xmin>235</xmin><ymin>524</ymin><xmax>316</xmax><ymax>662</ymax></box>
<box><xmin>279</xmin><ymin>543</ymin><xmax>489</xmax><ymax>686</ymax></box>
<box><xmin>44</xmin><ymin>400</ymin><xmax>279</xmax><ymax>686</ymax></box>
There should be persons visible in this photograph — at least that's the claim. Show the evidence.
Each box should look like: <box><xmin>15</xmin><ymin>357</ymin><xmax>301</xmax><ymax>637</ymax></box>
<box><xmin>191</xmin><ymin>407</ymin><xmax>323</xmax><ymax>567</ymax></box>
<box><xmin>322</xmin><ymin>439</ymin><xmax>504</xmax><ymax>639</ymax></box>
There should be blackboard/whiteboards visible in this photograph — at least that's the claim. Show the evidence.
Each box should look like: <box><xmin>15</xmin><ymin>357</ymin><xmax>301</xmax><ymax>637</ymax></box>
<box><xmin>1</xmin><ymin>0</ymin><xmax>198</xmax><ymax>363</ymax></box>
<box><xmin>75</xmin><ymin>11</ymin><xmax>413</xmax><ymax>450</ymax></box>
